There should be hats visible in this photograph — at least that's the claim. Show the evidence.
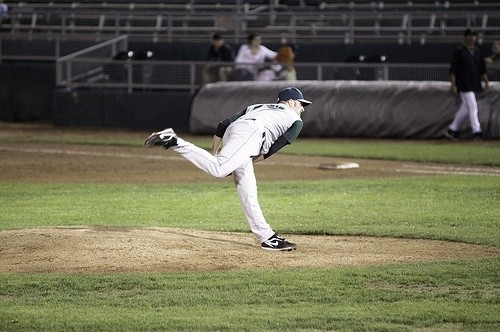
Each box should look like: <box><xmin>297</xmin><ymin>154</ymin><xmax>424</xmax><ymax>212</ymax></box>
<box><xmin>277</xmin><ymin>87</ymin><xmax>312</xmax><ymax>107</ymax></box>
<box><xmin>464</xmin><ymin>28</ymin><xmax>477</xmax><ymax>36</ymax></box>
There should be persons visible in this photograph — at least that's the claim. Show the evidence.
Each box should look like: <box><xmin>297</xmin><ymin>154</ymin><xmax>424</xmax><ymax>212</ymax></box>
<box><xmin>144</xmin><ymin>87</ymin><xmax>312</xmax><ymax>251</ymax></box>
<box><xmin>233</xmin><ymin>31</ymin><xmax>279</xmax><ymax>81</ymax></box>
<box><xmin>202</xmin><ymin>34</ymin><xmax>235</xmax><ymax>83</ymax></box>
<box><xmin>258</xmin><ymin>45</ymin><xmax>296</xmax><ymax>83</ymax></box>
<box><xmin>483</xmin><ymin>39</ymin><xmax>500</xmax><ymax>82</ymax></box>
<box><xmin>444</xmin><ymin>28</ymin><xmax>488</xmax><ymax>141</ymax></box>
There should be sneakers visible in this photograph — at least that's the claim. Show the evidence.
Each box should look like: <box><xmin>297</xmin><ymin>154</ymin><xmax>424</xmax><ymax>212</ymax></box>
<box><xmin>261</xmin><ymin>233</ymin><xmax>298</xmax><ymax>251</ymax></box>
<box><xmin>144</xmin><ymin>128</ymin><xmax>176</xmax><ymax>149</ymax></box>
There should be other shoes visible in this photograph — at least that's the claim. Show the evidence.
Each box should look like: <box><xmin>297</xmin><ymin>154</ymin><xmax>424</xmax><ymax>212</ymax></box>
<box><xmin>473</xmin><ymin>131</ymin><xmax>487</xmax><ymax>140</ymax></box>
<box><xmin>446</xmin><ymin>130</ymin><xmax>460</xmax><ymax>141</ymax></box>
